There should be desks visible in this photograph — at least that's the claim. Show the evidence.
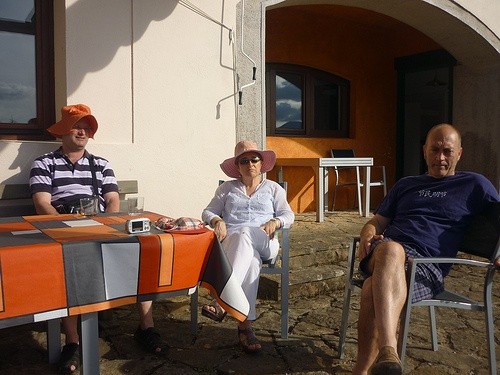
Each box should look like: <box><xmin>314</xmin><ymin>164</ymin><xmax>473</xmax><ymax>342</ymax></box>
<box><xmin>275</xmin><ymin>158</ymin><xmax>373</xmax><ymax>222</ymax></box>
<box><xmin>0</xmin><ymin>210</ymin><xmax>250</xmax><ymax>375</ymax></box>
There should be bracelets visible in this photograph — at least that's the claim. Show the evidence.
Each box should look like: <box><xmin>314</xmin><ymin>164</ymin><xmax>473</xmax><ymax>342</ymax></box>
<box><xmin>215</xmin><ymin>220</ymin><xmax>221</xmax><ymax>225</ymax></box>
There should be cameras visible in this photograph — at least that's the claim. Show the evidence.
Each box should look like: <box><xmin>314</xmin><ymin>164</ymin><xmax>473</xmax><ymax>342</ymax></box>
<box><xmin>125</xmin><ymin>218</ymin><xmax>151</xmax><ymax>233</ymax></box>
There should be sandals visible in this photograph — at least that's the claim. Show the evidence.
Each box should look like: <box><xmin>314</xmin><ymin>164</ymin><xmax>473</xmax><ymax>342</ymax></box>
<box><xmin>134</xmin><ymin>324</ymin><xmax>170</xmax><ymax>355</ymax></box>
<box><xmin>56</xmin><ymin>342</ymin><xmax>80</xmax><ymax>373</ymax></box>
<box><xmin>237</xmin><ymin>326</ymin><xmax>263</xmax><ymax>352</ymax></box>
<box><xmin>201</xmin><ymin>300</ymin><xmax>227</xmax><ymax>321</ymax></box>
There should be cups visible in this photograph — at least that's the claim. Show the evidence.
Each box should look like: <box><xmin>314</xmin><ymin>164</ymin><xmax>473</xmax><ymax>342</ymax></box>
<box><xmin>79</xmin><ymin>197</ymin><xmax>98</xmax><ymax>217</ymax></box>
<box><xmin>128</xmin><ymin>196</ymin><xmax>144</xmax><ymax>216</ymax></box>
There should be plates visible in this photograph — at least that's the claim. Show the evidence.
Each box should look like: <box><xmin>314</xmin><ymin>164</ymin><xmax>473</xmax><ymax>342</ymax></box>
<box><xmin>166</xmin><ymin>227</ymin><xmax>209</xmax><ymax>234</ymax></box>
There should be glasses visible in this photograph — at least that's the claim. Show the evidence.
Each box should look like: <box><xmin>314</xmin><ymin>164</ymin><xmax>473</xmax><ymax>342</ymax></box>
<box><xmin>236</xmin><ymin>156</ymin><xmax>262</xmax><ymax>165</ymax></box>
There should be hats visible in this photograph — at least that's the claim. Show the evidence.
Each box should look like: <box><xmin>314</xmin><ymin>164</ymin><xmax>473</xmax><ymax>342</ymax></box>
<box><xmin>47</xmin><ymin>104</ymin><xmax>98</xmax><ymax>138</ymax></box>
<box><xmin>220</xmin><ymin>141</ymin><xmax>276</xmax><ymax>178</ymax></box>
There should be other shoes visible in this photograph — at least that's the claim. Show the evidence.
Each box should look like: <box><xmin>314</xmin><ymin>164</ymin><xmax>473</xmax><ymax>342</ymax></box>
<box><xmin>371</xmin><ymin>346</ymin><xmax>403</xmax><ymax>375</ymax></box>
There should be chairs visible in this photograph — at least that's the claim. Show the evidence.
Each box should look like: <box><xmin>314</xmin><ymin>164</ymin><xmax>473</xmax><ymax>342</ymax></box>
<box><xmin>191</xmin><ymin>179</ymin><xmax>288</xmax><ymax>339</ymax></box>
<box><xmin>338</xmin><ymin>237</ymin><xmax>500</xmax><ymax>375</ymax></box>
<box><xmin>331</xmin><ymin>148</ymin><xmax>386</xmax><ymax>216</ymax></box>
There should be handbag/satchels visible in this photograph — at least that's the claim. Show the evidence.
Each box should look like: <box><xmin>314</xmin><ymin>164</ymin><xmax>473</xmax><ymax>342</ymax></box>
<box><xmin>56</xmin><ymin>195</ymin><xmax>100</xmax><ymax>215</ymax></box>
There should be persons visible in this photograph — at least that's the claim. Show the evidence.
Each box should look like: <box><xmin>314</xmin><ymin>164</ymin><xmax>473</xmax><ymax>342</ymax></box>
<box><xmin>29</xmin><ymin>104</ymin><xmax>165</xmax><ymax>375</ymax></box>
<box><xmin>352</xmin><ymin>123</ymin><xmax>500</xmax><ymax>375</ymax></box>
<box><xmin>202</xmin><ymin>139</ymin><xmax>295</xmax><ymax>354</ymax></box>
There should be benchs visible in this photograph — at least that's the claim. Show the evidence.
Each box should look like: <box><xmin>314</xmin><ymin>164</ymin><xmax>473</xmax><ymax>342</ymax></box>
<box><xmin>5</xmin><ymin>181</ymin><xmax>138</xmax><ymax>216</ymax></box>
